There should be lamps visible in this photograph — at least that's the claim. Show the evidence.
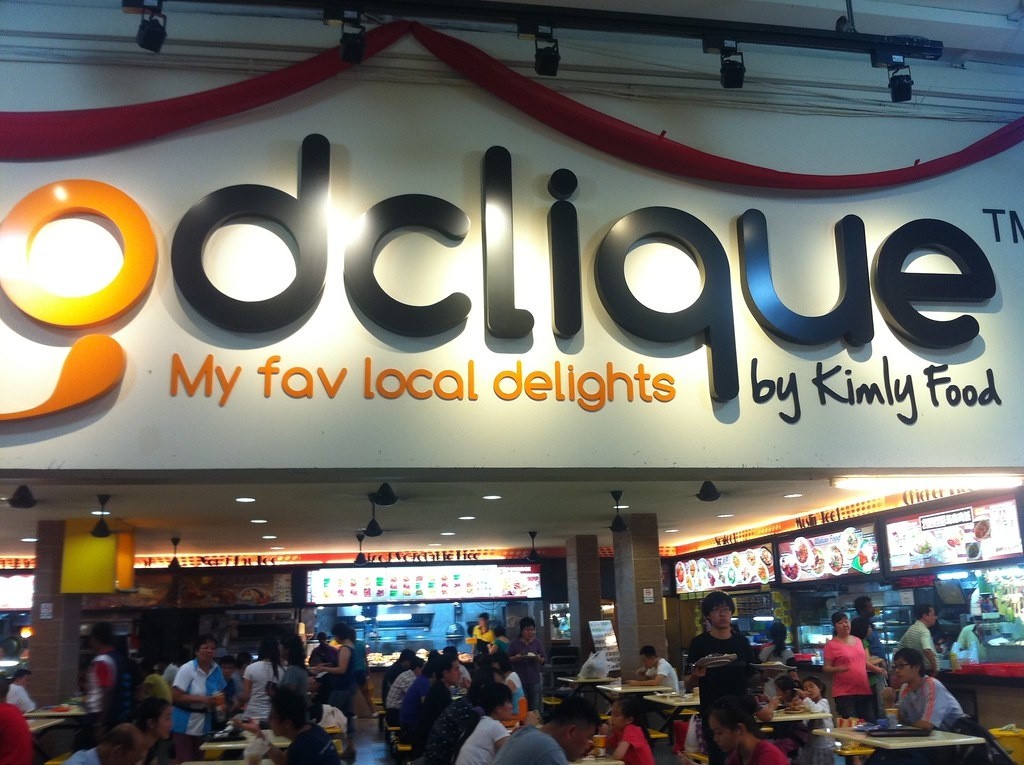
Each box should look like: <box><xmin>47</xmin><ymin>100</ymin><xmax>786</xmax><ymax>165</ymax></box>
<box><xmin>90</xmin><ymin>495</ymin><xmax>111</xmax><ymax>537</ymax></box>
<box><xmin>523</xmin><ymin>531</ymin><xmax>542</xmax><ymax>562</ymax></box>
<box><xmin>365</xmin><ymin>507</ymin><xmax>384</xmax><ymax>537</ymax></box>
<box><xmin>354</xmin><ymin>534</ymin><xmax>369</xmax><ymax>565</ymax></box>
<box><xmin>533</xmin><ymin>35</ymin><xmax>560</xmax><ymax>76</ymax></box>
<box><xmin>888</xmin><ymin>64</ymin><xmax>916</xmax><ymax>105</ymax></box>
<box><xmin>696</xmin><ymin>480</ymin><xmax>721</xmax><ymax>501</ymax></box>
<box><xmin>136</xmin><ymin>4</ymin><xmax>168</xmax><ymax>54</ymax></box>
<box><xmin>339</xmin><ymin>9</ymin><xmax>365</xmax><ymax>64</ymax></box>
<box><xmin>167</xmin><ymin>539</ymin><xmax>183</xmax><ymax>572</ymax></box>
<box><xmin>716</xmin><ymin>46</ymin><xmax>748</xmax><ymax>91</ymax></box>
<box><xmin>369</xmin><ymin>483</ymin><xmax>398</xmax><ymax>506</ymax></box>
<box><xmin>0</xmin><ymin>482</ymin><xmax>37</xmax><ymax>509</ymax></box>
<box><xmin>606</xmin><ymin>489</ymin><xmax>630</xmax><ymax>533</ymax></box>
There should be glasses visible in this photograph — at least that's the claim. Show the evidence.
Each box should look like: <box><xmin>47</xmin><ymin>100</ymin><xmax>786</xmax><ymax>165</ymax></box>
<box><xmin>890</xmin><ymin>662</ymin><xmax>911</xmax><ymax>669</ymax></box>
<box><xmin>710</xmin><ymin>606</ymin><xmax>732</xmax><ymax>614</ymax></box>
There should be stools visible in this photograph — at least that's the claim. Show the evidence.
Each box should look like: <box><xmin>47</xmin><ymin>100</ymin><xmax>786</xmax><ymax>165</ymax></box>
<box><xmin>47</xmin><ymin>694</ymin><xmax>876</xmax><ymax>765</ymax></box>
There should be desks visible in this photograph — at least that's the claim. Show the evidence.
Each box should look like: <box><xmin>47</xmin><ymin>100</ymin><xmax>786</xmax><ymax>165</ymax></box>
<box><xmin>507</xmin><ymin>678</ymin><xmax>984</xmax><ymax>765</ymax></box>
<box><xmin>25</xmin><ymin>695</ymin><xmax>341</xmax><ymax>765</ymax></box>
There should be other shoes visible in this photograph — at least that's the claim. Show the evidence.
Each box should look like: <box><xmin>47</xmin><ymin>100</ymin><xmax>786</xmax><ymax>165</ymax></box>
<box><xmin>340</xmin><ymin>748</ymin><xmax>357</xmax><ymax>763</ymax></box>
<box><xmin>354</xmin><ymin>715</ymin><xmax>358</xmax><ymax>719</ymax></box>
<box><xmin>371</xmin><ymin>712</ymin><xmax>379</xmax><ymax>718</ymax></box>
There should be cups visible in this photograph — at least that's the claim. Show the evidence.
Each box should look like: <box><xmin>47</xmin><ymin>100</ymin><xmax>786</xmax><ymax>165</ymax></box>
<box><xmin>884</xmin><ymin>708</ymin><xmax>898</xmax><ymax>728</ymax></box>
<box><xmin>592</xmin><ymin>735</ymin><xmax>608</xmax><ymax>760</ymax></box>
<box><xmin>949</xmin><ymin>653</ymin><xmax>962</xmax><ymax>670</ymax></box>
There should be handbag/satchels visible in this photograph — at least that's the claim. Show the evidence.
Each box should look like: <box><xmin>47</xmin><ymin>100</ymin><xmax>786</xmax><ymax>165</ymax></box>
<box><xmin>685</xmin><ymin>713</ymin><xmax>708</xmax><ymax>754</ymax></box>
<box><xmin>672</xmin><ymin>719</ymin><xmax>688</xmax><ymax>752</ymax></box>
<box><xmin>951</xmin><ymin>641</ymin><xmax>978</xmax><ymax>665</ymax></box>
<box><xmin>580</xmin><ymin>649</ymin><xmax>612</xmax><ymax>680</ymax></box>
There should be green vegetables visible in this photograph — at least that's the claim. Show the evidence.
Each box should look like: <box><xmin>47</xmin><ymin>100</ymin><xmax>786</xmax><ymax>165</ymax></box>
<box><xmin>832</xmin><ymin>535</ymin><xmax>853</xmax><ymax>567</ymax></box>
<box><xmin>969</xmin><ymin>542</ymin><xmax>979</xmax><ymax>558</ymax></box>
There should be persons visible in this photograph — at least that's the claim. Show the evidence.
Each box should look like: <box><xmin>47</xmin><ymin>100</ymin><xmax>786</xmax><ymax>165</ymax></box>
<box><xmin>958</xmin><ymin>617</ymin><xmax>1002</xmax><ymax>661</ymax></box>
<box><xmin>0</xmin><ymin>669</ymin><xmax>39</xmax><ymax>765</ymax></box>
<box><xmin>383</xmin><ymin>611</ymin><xmax>525</xmax><ymax>765</ymax></box>
<box><xmin>598</xmin><ymin>697</ymin><xmax>656</xmax><ymax>765</ymax></box>
<box><xmin>507</xmin><ymin>618</ymin><xmax>544</xmax><ymax>710</ymax></box>
<box><xmin>854</xmin><ymin>596</ymin><xmax>886</xmax><ymax>659</ymax></box>
<box><xmin>897</xmin><ymin>602</ymin><xmax>939</xmax><ymax>676</ymax></box>
<box><xmin>628</xmin><ymin>646</ymin><xmax>679</xmax><ymax>729</ymax></box>
<box><xmin>823</xmin><ymin>612</ymin><xmax>888</xmax><ymax>718</ymax></box>
<box><xmin>57</xmin><ymin>621</ymin><xmax>227</xmax><ymax>765</ymax></box>
<box><xmin>708</xmin><ymin>692</ymin><xmax>790</xmax><ymax>765</ymax></box>
<box><xmin>758</xmin><ymin>624</ymin><xmax>798</xmax><ymax>698</ymax></box>
<box><xmin>234</xmin><ymin>691</ymin><xmax>342</xmax><ymax>765</ymax></box>
<box><xmin>851</xmin><ymin>619</ymin><xmax>887</xmax><ymax>715</ymax></box>
<box><xmin>310</xmin><ymin>623</ymin><xmax>380</xmax><ymax>759</ymax></box>
<box><xmin>882</xmin><ymin>648</ymin><xmax>964</xmax><ymax>732</ymax></box>
<box><xmin>684</xmin><ymin>591</ymin><xmax>780</xmax><ymax>765</ymax></box>
<box><xmin>759</xmin><ymin>675</ymin><xmax>835</xmax><ymax>765</ymax></box>
<box><xmin>493</xmin><ymin>696</ymin><xmax>602</xmax><ymax>765</ymax></box>
<box><xmin>219</xmin><ymin>633</ymin><xmax>317</xmax><ymax>731</ymax></box>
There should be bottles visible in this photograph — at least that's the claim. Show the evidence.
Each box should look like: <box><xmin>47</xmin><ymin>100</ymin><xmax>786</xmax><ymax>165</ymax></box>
<box><xmin>243</xmin><ymin>751</ymin><xmax>263</xmax><ymax>765</ymax></box>
<box><xmin>213</xmin><ymin>692</ymin><xmax>226</xmax><ymax>710</ymax></box>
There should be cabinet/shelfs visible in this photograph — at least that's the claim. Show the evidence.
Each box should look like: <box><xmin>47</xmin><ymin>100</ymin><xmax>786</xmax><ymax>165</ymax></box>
<box><xmin>872</xmin><ymin>605</ymin><xmax>914</xmax><ymax>664</ymax></box>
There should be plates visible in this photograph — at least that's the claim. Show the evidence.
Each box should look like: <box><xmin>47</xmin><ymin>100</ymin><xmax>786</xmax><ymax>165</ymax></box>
<box><xmin>239</xmin><ymin>587</ymin><xmax>273</xmax><ymax>605</ymax></box>
<box><xmin>694</xmin><ymin>653</ymin><xmax>738</xmax><ymax>668</ymax></box>
<box><xmin>785</xmin><ymin>710</ymin><xmax>805</xmax><ymax>713</ymax></box>
<box><xmin>885</xmin><ymin>512</ymin><xmax>997</xmax><ymax>566</ymax></box>
<box><xmin>208</xmin><ymin>588</ymin><xmax>239</xmax><ymax>608</ymax></box>
<box><xmin>749</xmin><ymin>662</ymin><xmax>797</xmax><ymax>671</ymax></box>
<box><xmin>675</xmin><ymin>525</ymin><xmax>882</xmax><ymax>592</ymax></box>
<box><xmin>520</xmin><ymin>655</ymin><xmax>538</xmax><ymax>658</ymax></box>
